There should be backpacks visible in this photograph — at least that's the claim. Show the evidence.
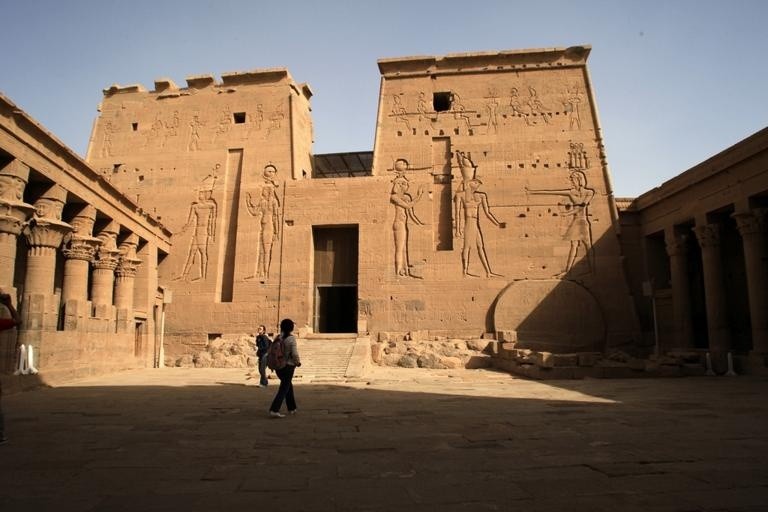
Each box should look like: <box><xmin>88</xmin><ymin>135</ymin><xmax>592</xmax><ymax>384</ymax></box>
<box><xmin>267</xmin><ymin>333</ymin><xmax>294</xmax><ymax>370</ymax></box>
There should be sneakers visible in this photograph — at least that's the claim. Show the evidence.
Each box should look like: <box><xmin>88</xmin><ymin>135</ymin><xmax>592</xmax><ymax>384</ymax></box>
<box><xmin>269</xmin><ymin>408</ymin><xmax>297</xmax><ymax>418</ymax></box>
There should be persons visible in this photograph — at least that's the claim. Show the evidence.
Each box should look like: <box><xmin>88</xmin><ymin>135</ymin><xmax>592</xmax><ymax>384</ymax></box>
<box><xmin>0</xmin><ymin>288</ymin><xmax>22</xmax><ymax>447</ymax></box>
<box><xmin>254</xmin><ymin>325</ymin><xmax>271</xmax><ymax>388</ymax></box>
<box><xmin>269</xmin><ymin>318</ymin><xmax>302</xmax><ymax>418</ymax></box>
